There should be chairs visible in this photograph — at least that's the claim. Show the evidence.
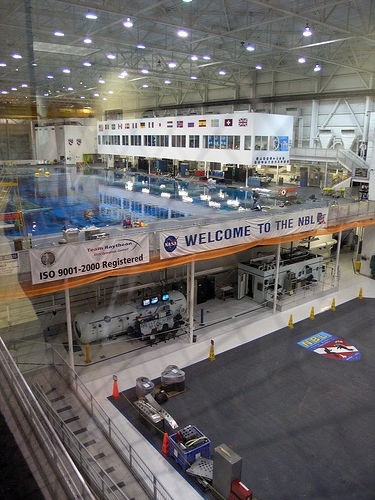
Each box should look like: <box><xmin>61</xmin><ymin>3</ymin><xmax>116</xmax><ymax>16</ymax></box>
<box><xmin>127</xmin><ymin>313</ymin><xmax>184</xmax><ymax>347</ymax></box>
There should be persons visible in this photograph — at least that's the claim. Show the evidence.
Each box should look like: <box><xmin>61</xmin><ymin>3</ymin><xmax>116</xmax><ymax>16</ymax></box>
<box><xmin>359</xmin><ymin>141</ymin><xmax>366</xmax><ymax>158</ymax></box>
<box><xmin>331</xmin><ymin>200</ymin><xmax>338</xmax><ymax>208</ymax></box>
<box><xmin>135</xmin><ymin>316</ymin><xmax>143</xmax><ymax>340</ymax></box>
<box><xmin>126</xmin><ymin>217</ymin><xmax>133</xmax><ymax>228</ymax></box>
<box><xmin>159</xmin><ymin>317</ymin><xmax>180</xmax><ymax>342</ymax></box>
<box><xmin>252</xmin><ymin>190</ymin><xmax>260</xmax><ymax>209</ymax></box>
<box><xmin>157</xmin><ymin>170</ymin><xmax>161</xmax><ymax>175</ymax></box>
<box><xmin>274</xmin><ymin>137</ymin><xmax>278</xmax><ymax>149</ymax></box>
<box><xmin>211</xmin><ymin>170</ymin><xmax>214</xmax><ymax>175</ymax></box>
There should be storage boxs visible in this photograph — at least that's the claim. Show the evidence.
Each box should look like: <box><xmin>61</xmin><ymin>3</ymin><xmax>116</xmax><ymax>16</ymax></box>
<box><xmin>168</xmin><ymin>424</ymin><xmax>211</xmax><ymax>471</ymax></box>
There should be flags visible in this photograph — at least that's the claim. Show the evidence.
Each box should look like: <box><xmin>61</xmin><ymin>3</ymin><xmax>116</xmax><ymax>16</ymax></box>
<box><xmin>177</xmin><ymin>121</ymin><xmax>184</xmax><ymax>127</ymax></box>
<box><xmin>211</xmin><ymin>119</ymin><xmax>219</xmax><ymax>127</ymax></box>
<box><xmin>225</xmin><ymin>119</ymin><xmax>232</xmax><ymax>126</ymax></box>
<box><xmin>188</xmin><ymin>121</ymin><xmax>194</xmax><ymax>127</ymax></box>
<box><xmin>167</xmin><ymin>120</ymin><xmax>173</xmax><ymax>127</ymax></box>
<box><xmin>199</xmin><ymin>120</ymin><xmax>206</xmax><ymax>126</ymax></box>
<box><xmin>239</xmin><ymin>118</ymin><xmax>248</xmax><ymax>126</ymax></box>
<box><xmin>99</xmin><ymin>122</ymin><xmax>164</xmax><ymax>131</ymax></box>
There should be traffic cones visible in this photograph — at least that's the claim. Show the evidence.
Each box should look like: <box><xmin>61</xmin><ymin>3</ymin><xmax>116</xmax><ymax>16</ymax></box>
<box><xmin>209</xmin><ymin>339</ymin><xmax>216</xmax><ymax>362</ymax></box>
<box><xmin>287</xmin><ymin>314</ymin><xmax>294</xmax><ymax>329</ymax></box>
<box><xmin>358</xmin><ymin>287</ymin><xmax>363</xmax><ymax>300</ymax></box>
<box><xmin>161</xmin><ymin>432</ymin><xmax>170</xmax><ymax>457</ymax></box>
<box><xmin>111</xmin><ymin>374</ymin><xmax>121</xmax><ymax>399</ymax></box>
<box><xmin>309</xmin><ymin>305</ymin><xmax>315</xmax><ymax>321</ymax></box>
<box><xmin>330</xmin><ymin>298</ymin><xmax>336</xmax><ymax>312</ymax></box>
